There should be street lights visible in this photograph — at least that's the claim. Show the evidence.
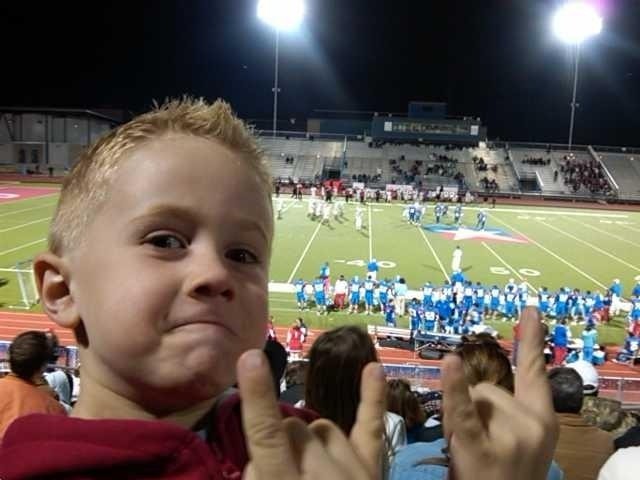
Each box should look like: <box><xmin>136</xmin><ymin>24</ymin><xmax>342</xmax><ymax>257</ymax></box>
<box><xmin>258</xmin><ymin>0</ymin><xmax>303</xmax><ymax>137</ymax></box>
<box><xmin>552</xmin><ymin>3</ymin><xmax>601</xmax><ymax>151</ymax></box>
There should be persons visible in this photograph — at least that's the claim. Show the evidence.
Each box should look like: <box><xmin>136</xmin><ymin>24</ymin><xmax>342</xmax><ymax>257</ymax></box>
<box><xmin>1</xmin><ymin>244</ymin><xmax>638</xmax><ymax>479</ymax></box>
<box><xmin>0</xmin><ymin>91</ymin><xmax>561</xmax><ymax>480</ymax></box>
<box><xmin>271</xmin><ymin>120</ymin><xmax>617</xmax><ymax>231</ymax></box>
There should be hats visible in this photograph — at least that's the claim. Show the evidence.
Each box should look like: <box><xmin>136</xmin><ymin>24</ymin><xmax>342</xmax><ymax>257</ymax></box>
<box><xmin>564</xmin><ymin>360</ymin><xmax>600</xmax><ymax>395</ymax></box>
